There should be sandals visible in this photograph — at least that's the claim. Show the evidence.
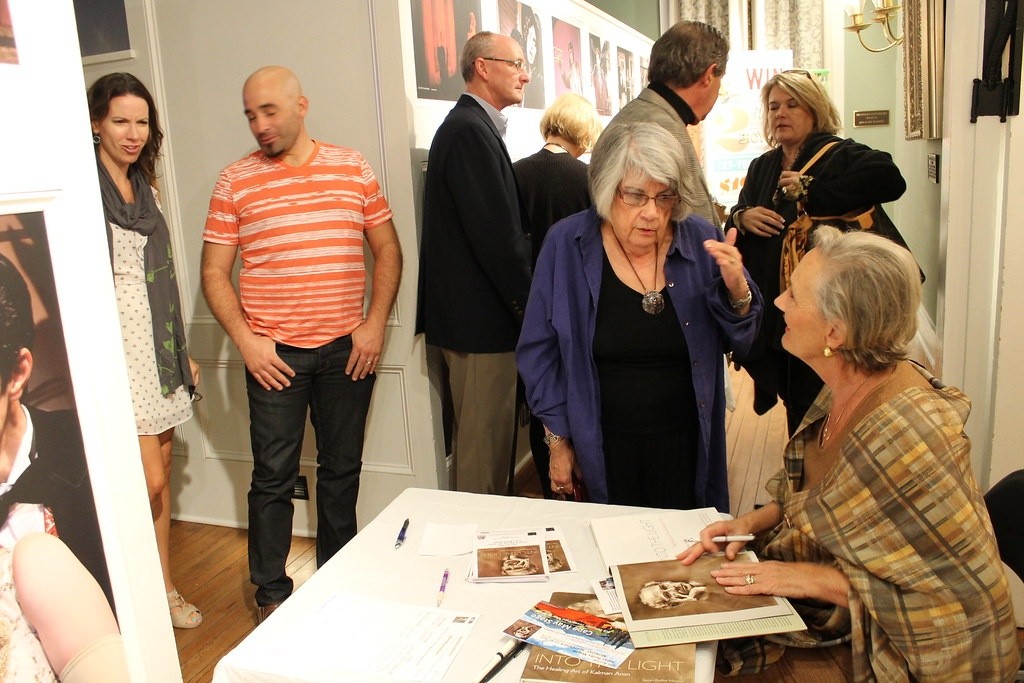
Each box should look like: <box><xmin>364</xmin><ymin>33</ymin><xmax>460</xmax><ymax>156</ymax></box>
<box><xmin>166</xmin><ymin>587</ymin><xmax>203</xmax><ymax>628</ymax></box>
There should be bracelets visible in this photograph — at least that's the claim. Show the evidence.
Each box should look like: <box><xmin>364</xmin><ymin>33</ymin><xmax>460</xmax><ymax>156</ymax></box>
<box><xmin>728</xmin><ymin>287</ymin><xmax>754</xmax><ymax>310</ymax></box>
<box><xmin>542</xmin><ymin>432</ymin><xmax>566</xmax><ymax>456</ymax></box>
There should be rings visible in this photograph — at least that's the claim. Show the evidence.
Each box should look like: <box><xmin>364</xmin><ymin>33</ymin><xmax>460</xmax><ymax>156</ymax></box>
<box><xmin>366</xmin><ymin>362</ymin><xmax>373</xmax><ymax>365</ymax></box>
<box><xmin>728</xmin><ymin>255</ymin><xmax>736</xmax><ymax>265</ymax></box>
<box><xmin>556</xmin><ymin>485</ymin><xmax>565</xmax><ymax>490</ymax></box>
<box><xmin>747</xmin><ymin>574</ymin><xmax>754</xmax><ymax>586</ymax></box>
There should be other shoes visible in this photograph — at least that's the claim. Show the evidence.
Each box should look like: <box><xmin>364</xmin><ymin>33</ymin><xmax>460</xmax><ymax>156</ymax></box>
<box><xmin>252</xmin><ymin>596</ymin><xmax>282</xmax><ymax>625</ymax></box>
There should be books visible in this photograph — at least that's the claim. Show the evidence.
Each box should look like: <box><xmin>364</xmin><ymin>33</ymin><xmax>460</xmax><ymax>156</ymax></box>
<box><xmin>587</xmin><ymin>505</ymin><xmax>809</xmax><ymax>651</ymax></box>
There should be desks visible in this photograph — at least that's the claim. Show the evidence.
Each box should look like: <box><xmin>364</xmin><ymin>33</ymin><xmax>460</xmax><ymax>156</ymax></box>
<box><xmin>211</xmin><ymin>486</ymin><xmax>737</xmax><ymax>682</ymax></box>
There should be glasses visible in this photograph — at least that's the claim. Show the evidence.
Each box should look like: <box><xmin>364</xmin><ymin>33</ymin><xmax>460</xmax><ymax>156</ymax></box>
<box><xmin>471</xmin><ymin>57</ymin><xmax>525</xmax><ymax>73</ymax></box>
<box><xmin>782</xmin><ymin>70</ymin><xmax>820</xmax><ymax>91</ymax></box>
<box><xmin>616</xmin><ymin>186</ymin><xmax>679</xmax><ymax>209</ymax></box>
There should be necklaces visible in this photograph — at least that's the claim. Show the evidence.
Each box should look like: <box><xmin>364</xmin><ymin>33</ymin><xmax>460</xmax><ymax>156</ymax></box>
<box><xmin>541</xmin><ymin>142</ymin><xmax>572</xmax><ymax>154</ymax></box>
<box><xmin>818</xmin><ymin>369</ymin><xmax>877</xmax><ymax>450</ymax></box>
<box><xmin>607</xmin><ymin>222</ymin><xmax>665</xmax><ymax>315</ymax></box>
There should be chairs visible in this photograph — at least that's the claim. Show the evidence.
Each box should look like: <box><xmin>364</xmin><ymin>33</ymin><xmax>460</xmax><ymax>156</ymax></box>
<box><xmin>982</xmin><ymin>469</ymin><xmax>1024</xmax><ymax>584</ymax></box>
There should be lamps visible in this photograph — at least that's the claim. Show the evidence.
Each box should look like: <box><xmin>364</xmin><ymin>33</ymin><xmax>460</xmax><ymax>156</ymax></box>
<box><xmin>841</xmin><ymin>0</ymin><xmax>905</xmax><ymax>52</ymax></box>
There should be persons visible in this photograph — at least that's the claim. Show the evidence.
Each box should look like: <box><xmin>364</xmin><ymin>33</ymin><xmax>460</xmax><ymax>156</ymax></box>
<box><xmin>414</xmin><ymin>31</ymin><xmax>534</xmax><ymax>496</ymax></box>
<box><xmin>512</xmin><ymin>89</ymin><xmax>601</xmax><ymax>503</ymax></box>
<box><xmin>515</xmin><ymin>119</ymin><xmax>766</xmax><ymax>514</ymax></box>
<box><xmin>588</xmin><ymin>20</ymin><xmax>727</xmax><ymax>230</ymax></box>
<box><xmin>724</xmin><ymin>69</ymin><xmax>926</xmax><ymax>441</ymax></box>
<box><xmin>675</xmin><ymin>226</ymin><xmax>1024</xmax><ymax>683</ymax></box>
<box><xmin>87</xmin><ymin>72</ymin><xmax>203</xmax><ymax>629</ymax></box>
<box><xmin>0</xmin><ymin>252</ymin><xmax>149</xmax><ymax>683</ymax></box>
<box><xmin>199</xmin><ymin>70</ymin><xmax>404</xmax><ymax>626</ymax></box>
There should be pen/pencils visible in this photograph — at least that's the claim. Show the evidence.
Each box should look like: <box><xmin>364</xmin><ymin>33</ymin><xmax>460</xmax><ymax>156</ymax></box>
<box><xmin>684</xmin><ymin>534</ymin><xmax>755</xmax><ymax>543</ymax></box>
<box><xmin>478</xmin><ymin>642</ymin><xmax>527</xmax><ymax>683</ymax></box>
<box><xmin>395</xmin><ymin>518</ymin><xmax>409</xmax><ymax>549</ymax></box>
<box><xmin>438</xmin><ymin>570</ymin><xmax>449</xmax><ymax>606</ymax></box>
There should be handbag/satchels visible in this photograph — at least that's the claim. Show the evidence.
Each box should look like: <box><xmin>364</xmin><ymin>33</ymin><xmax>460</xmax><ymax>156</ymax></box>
<box><xmin>780</xmin><ymin>142</ymin><xmax>879</xmax><ymax>295</ymax></box>
